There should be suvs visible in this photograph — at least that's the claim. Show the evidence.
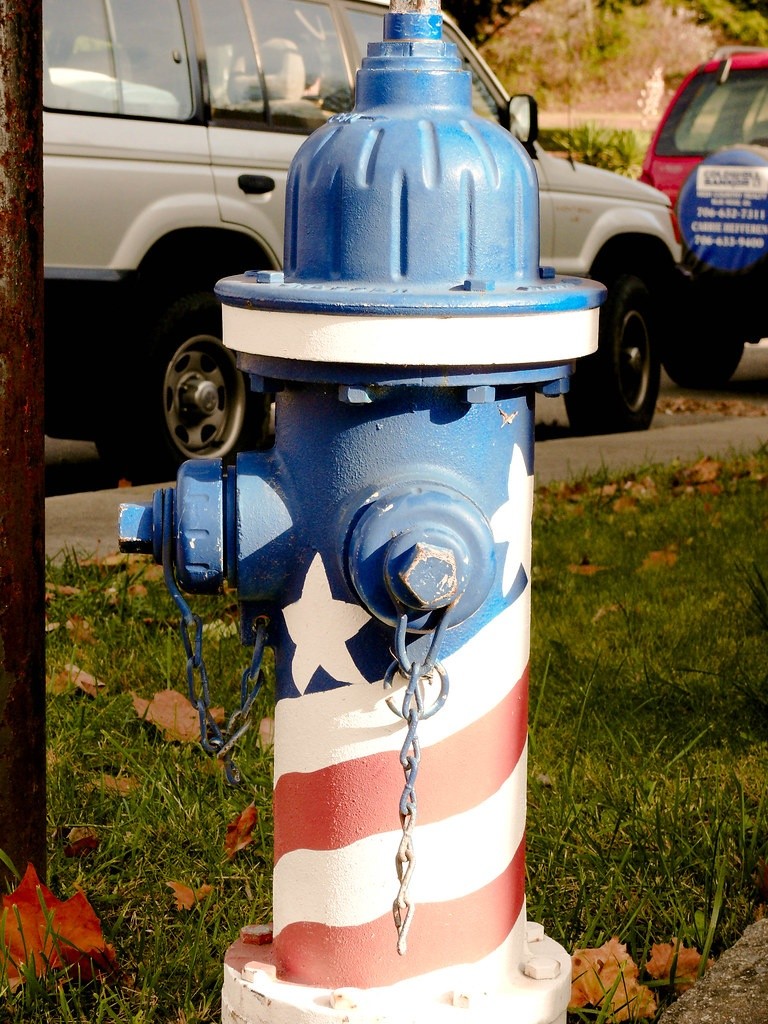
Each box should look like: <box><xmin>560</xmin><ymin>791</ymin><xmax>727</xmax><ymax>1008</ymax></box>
<box><xmin>638</xmin><ymin>45</ymin><xmax>768</xmax><ymax>389</ymax></box>
<box><xmin>44</xmin><ymin>0</ymin><xmax>691</xmax><ymax>488</ymax></box>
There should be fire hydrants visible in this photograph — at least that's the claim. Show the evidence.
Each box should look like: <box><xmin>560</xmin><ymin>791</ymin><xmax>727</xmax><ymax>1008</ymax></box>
<box><xmin>118</xmin><ymin>0</ymin><xmax>609</xmax><ymax>1024</ymax></box>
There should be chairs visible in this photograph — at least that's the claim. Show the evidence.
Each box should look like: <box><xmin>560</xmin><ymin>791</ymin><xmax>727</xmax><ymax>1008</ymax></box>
<box><xmin>225</xmin><ymin>38</ymin><xmax>306</xmax><ymax>101</ymax></box>
